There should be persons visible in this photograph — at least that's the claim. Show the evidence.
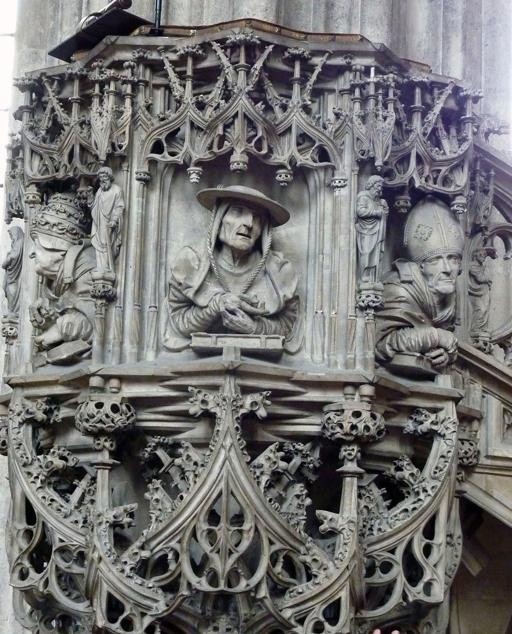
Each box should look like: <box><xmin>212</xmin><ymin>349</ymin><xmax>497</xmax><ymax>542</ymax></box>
<box><xmin>25</xmin><ymin>191</ymin><xmax>99</xmax><ymax>364</ymax></box>
<box><xmin>372</xmin><ymin>196</ymin><xmax>464</xmax><ymax>373</ymax></box>
<box><xmin>2</xmin><ymin>224</ymin><xmax>25</xmax><ymax>322</ymax></box>
<box><xmin>87</xmin><ymin>166</ymin><xmax>125</xmax><ymax>273</ymax></box>
<box><xmin>354</xmin><ymin>174</ymin><xmax>390</xmax><ymax>283</ymax></box>
<box><xmin>166</xmin><ymin>181</ymin><xmax>298</xmax><ymax>339</ymax></box>
<box><xmin>467</xmin><ymin>249</ymin><xmax>494</xmax><ymax>354</ymax></box>
